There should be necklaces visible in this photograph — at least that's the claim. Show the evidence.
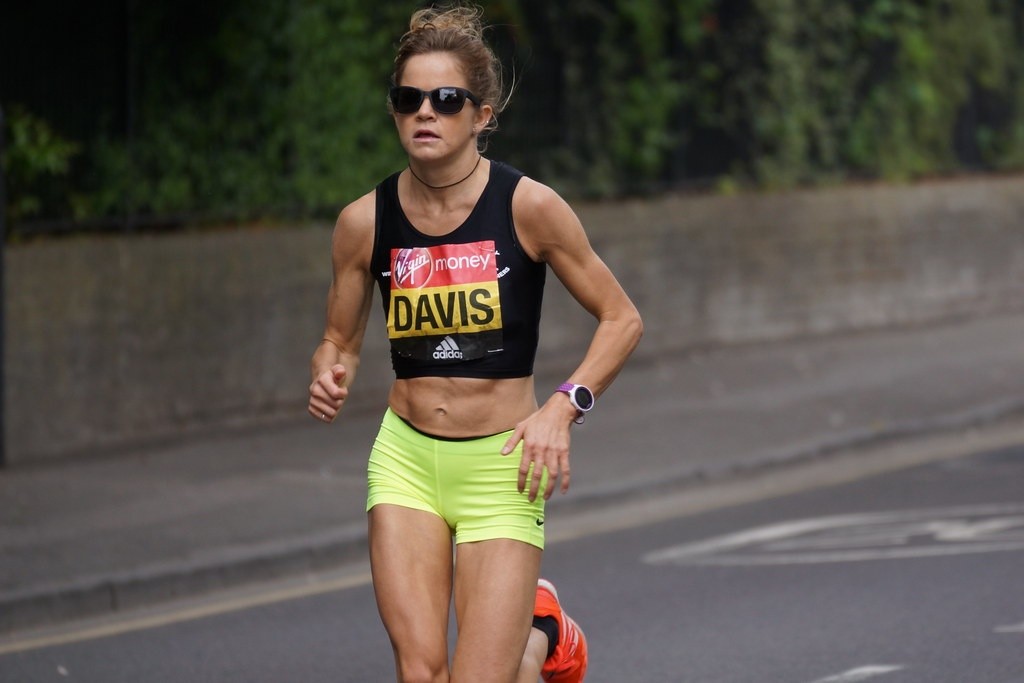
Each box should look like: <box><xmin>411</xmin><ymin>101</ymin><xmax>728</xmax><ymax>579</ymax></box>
<box><xmin>409</xmin><ymin>157</ymin><xmax>481</xmax><ymax>188</ymax></box>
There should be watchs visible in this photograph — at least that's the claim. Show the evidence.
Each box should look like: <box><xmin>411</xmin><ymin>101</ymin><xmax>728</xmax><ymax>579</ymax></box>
<box><xmin>556</xmin><ymin>382</ymin><xmax>594</xmax><ymax>424</ymax></box>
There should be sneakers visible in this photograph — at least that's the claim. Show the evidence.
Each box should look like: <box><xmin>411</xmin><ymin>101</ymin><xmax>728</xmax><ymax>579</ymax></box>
<box><xmin>533</xmin><ymin>577</ymin><xmax>588</xmax><ymax>683</ymax></box>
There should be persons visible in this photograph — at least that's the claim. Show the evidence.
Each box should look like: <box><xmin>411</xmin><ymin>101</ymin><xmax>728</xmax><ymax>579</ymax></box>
<box><xmin>307</xmin><ymin>8</ymin><xmax>643</xmax><ymax>683</ymax></box>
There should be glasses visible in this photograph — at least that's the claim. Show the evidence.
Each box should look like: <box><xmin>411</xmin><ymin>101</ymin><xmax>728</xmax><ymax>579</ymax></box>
<box><xmin>389</xmin><ymin>85</ymin><xmax>482</xmax><ymax>115</ymax></box>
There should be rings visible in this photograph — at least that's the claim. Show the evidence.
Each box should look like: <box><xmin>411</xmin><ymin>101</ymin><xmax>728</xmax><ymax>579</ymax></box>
<box><xmin>322</xmin><ymin>414</ymin><xmax>325</xmax><ymax>418</ymax></box>
